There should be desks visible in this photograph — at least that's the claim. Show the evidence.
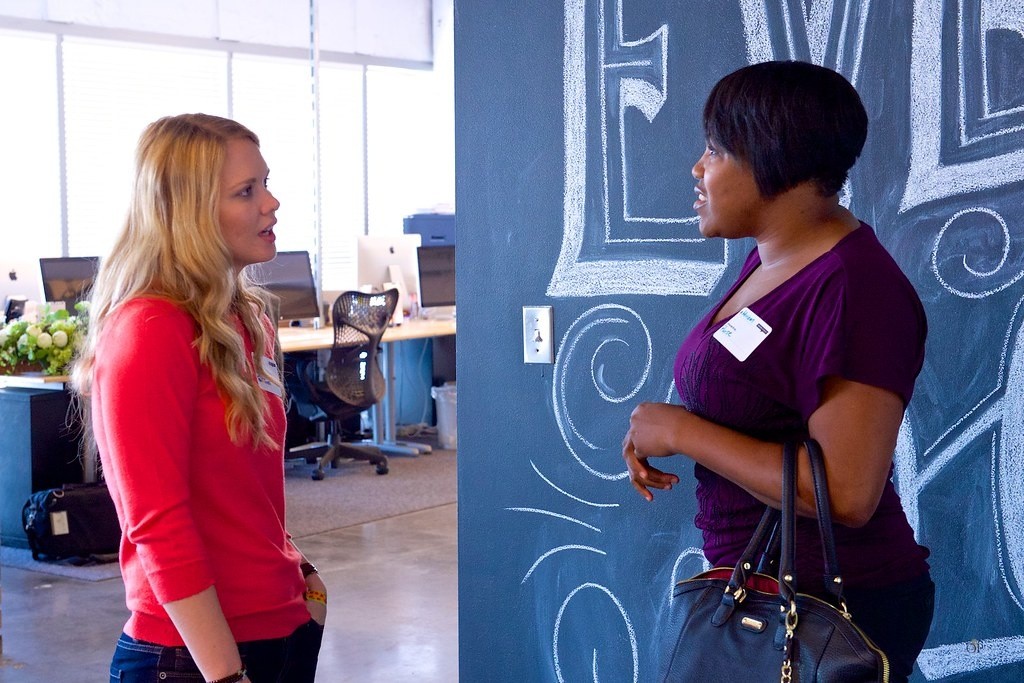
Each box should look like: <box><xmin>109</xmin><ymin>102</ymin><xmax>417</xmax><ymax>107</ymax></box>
<box><xmin>44</xmin><ymin>313</ymin><xmax>457</xmax><ymax>486</ymax></box>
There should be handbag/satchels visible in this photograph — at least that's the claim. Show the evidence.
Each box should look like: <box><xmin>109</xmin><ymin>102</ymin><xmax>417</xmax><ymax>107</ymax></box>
<box><xmin>655</xmin><ymin>438</ymin><xmax>895</xmax><ymax>683</ymax></box>
<box><xmin>21</xmin><ymin>482</ymin><xmax>122</xmax><ymax>566</ymax></box>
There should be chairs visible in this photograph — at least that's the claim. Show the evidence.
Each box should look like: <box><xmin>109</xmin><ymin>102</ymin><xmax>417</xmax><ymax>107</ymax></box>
<box><xmin>289</xmin><ymin>289</ymin><xmax>399</xmax><ymax>481</ymax></box>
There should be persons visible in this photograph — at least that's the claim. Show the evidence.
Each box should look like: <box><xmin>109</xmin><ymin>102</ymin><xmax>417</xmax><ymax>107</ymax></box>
<box><xmin>621</xmin><ymin>62</ymin><xmax>938</xmax><ymax>683</ymax></box>
<box><xmin>63</xmin><ymin>112</ymin><xmax>328</xmax><ymax>683</ymax></box>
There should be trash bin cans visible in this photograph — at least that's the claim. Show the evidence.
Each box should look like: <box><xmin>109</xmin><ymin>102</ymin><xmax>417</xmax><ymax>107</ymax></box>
<box><xmin>431</xmin><ymin>382</ymin><xmax>457</xmax><ymax>450</ymax></box>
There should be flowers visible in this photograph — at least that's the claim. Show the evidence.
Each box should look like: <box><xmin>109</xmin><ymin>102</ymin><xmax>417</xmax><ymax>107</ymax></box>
<box><xmin>0</xmin><ymin>301</ymin><xmax>91</xmax><ymax>376</ymax></box>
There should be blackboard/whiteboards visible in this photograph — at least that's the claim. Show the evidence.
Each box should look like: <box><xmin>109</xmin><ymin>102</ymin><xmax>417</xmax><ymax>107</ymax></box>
<box><xmin>454</xmin><ymin>1</ymin><xmax>1023</xmax><ymax>683</ymax></box>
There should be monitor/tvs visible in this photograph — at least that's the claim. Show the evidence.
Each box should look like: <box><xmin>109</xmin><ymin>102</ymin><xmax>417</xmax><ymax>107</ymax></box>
<box><xmin>356</xmin><ymin>233</ymin><xmax>422</xmax><ymax>311</ymax></box>
<box><xmin>244</xmin><ymin>251</ymin><xmax>320</xmax><ymax>320</ymax></box>
<box><xmin>0</xmin><ymin>250</ymin><xmax>44</xmax><ymax>324</ymax></box>
<box><xmin>417</xmin><ymin>245</ymin><xmax>456</xmax><ymax>307</ymax></box>
<box><xmin>39</xmin><ymin>256</ymin><xmax>100</xmax><ymax>314</ymax></box>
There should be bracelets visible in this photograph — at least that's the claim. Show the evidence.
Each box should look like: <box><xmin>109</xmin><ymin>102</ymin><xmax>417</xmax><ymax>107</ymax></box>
<box><xmin>300</xmin><ymin>563</ymin><xmax>326</xmax><ymax>606</ymax></box>
<box><xmin>205</xmin><ymin>667</ymin><xmax>247</xmax><ymax>683</ymax></box>
